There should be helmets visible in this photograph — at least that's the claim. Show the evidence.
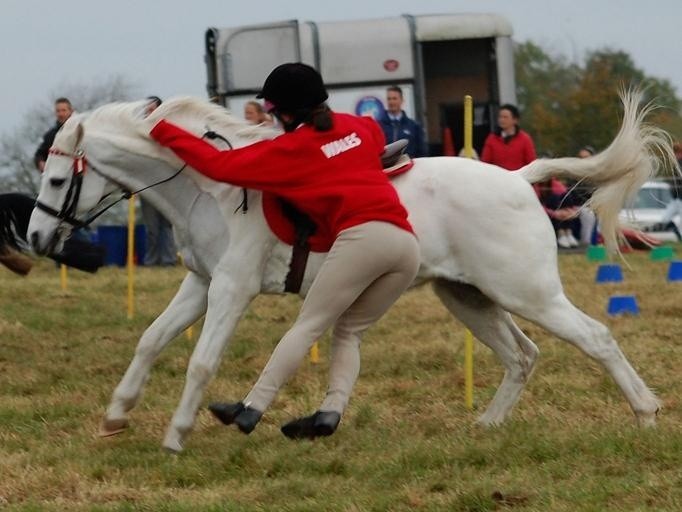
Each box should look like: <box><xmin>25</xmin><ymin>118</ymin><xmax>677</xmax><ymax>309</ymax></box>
<box><xmin>256</xmin><ymin>64</ymin><xmax>329</xmax><ymax>104</ymax></box>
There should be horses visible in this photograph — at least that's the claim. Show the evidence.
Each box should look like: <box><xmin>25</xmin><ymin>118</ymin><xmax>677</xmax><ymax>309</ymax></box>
<box><xmin>24</xmin><ymin>74</ymin><xmax>682</xmax><ymax>466</ymax></box>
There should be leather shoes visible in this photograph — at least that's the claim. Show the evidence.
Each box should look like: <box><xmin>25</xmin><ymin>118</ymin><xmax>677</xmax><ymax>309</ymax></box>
<box><xmin>209</xmin><ymin>402</ymin><xmax>263</xmax><ymax>436</ymax></box>
<box><xmin>281</xmin><ymin>411</ymin><xmax>340</xmax><ymax>438</ymax></box>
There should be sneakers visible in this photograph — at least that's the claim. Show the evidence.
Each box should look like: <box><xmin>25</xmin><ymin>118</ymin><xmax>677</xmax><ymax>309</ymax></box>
<box><xmin>558</xmin><ymin>235</ymin><xmax>578</xmax><ymax>248</ymax></box>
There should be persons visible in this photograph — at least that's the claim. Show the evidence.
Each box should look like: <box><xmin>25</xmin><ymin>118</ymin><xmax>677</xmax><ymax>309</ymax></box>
<box><xmin>139</xmin><ymin>96</ymin><xmax>178</xmax><ymax>267</ymax></box>
<box><xmin>376</xmin><ymin>87</ymin><xmax>425</xmax><ymax>158</ymax></box>
<box><xmin>34</xmin><ymin>98</ymin><xmax>74</xmax><ymax>174</ymax></box>
<box><xmin>533</xmin><ymin>144</ymin><xmax>598</xmax><ymax>251</ymax></box>
<box><xmin>245</xmin><ymin>100</ymin><xmax>266</xmax><ymax>124</ymax></box>
<box><xmin>479</xmin><ymin>104</ymin><xmax>541</xmax><ymax>197</ymax></box>
<box><xmin>149</xmin><ymin>62</ymin><xmax>422</xmax><ymax>439</ymax></box>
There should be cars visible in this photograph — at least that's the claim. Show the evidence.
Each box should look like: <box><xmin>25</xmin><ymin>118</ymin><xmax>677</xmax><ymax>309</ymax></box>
<box><xmin>619</xmin><ymin>180</ymin><xmax>682</xmax><ymax>232</ymax></box>
